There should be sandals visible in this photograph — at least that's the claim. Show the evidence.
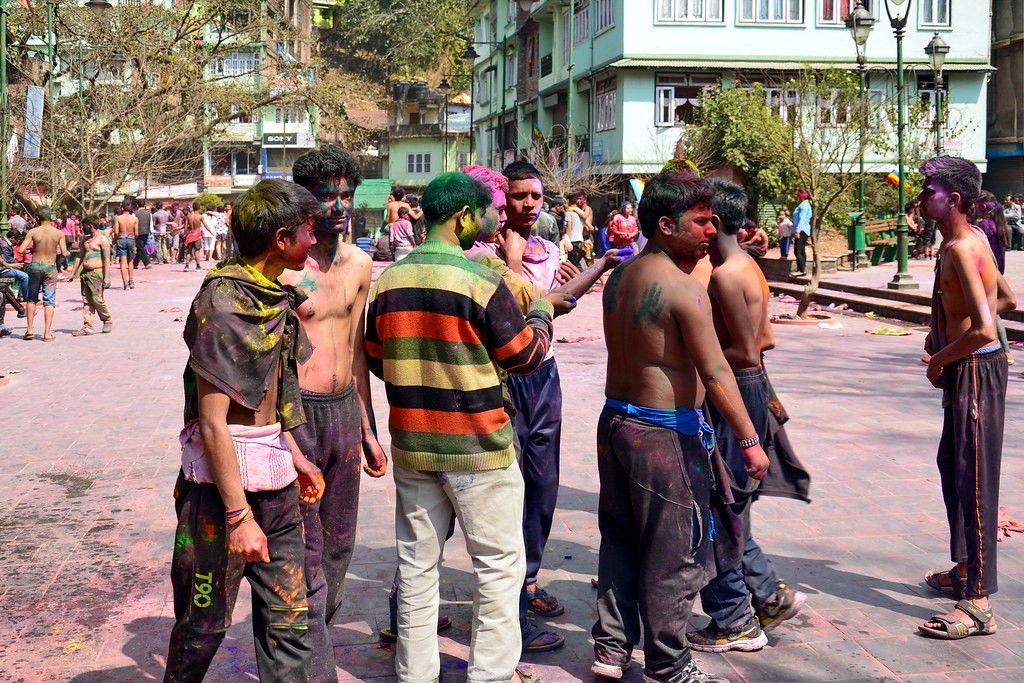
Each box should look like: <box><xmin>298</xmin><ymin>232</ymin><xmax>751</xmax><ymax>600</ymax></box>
<box><xmin>526</xmin><ymin>589</ymin><xmax>565</xmax><ymax>617</ymax></box>
<box><xmin>918</xmin><ymin>598</ymin><xmax>997</xmax><ymax>638</ymax></box>
<box><xmin>924</xmin><ymin>566</ymin><xmax>968</xmax><ymax>599</ymax></box>
<box><xmin>522</xmin><ymin>624</ymin><xmax>566</xmax><ymax>652</ymax></box>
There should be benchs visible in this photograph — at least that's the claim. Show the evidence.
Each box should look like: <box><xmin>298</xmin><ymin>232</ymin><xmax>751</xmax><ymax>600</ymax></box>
<box><xmin>792</xmin><ymin>257</ymin><xmax>838</xmax><ymax>272</ymax></box>
<box><xmin>865</xmin><ymin>218</ymin><xmax>920</xmax><ymax>265</ymax></box>
<box><xmin>822</xmin><ymin>250</ymin><xmax>857</xmax><ymax>271</ymax></box>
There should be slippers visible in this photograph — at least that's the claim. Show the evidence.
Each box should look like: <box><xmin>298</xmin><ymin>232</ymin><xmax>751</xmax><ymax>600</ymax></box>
<box><xmin>44</xmin><ymin>334</ymin><xmax>56</xmax><ymax>342</ymax></box>
<box><xmin>380</xmin><ymin>618</ymin><xmax>452</xmax><ymax>643</ymax></box>
<box><xmin>24</xmin><ymin>331</ymin><xmax>34</xmax><ymax>339</ymax></box>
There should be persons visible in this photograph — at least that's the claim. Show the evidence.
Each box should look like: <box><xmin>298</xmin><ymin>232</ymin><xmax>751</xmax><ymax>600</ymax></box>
<box><xmin>0</xmin><ymin>199</ymin><xmax>169</xmax><ymax>341</ymax></box>
<box><xmin>163</xmin><ymin>145</ymin><xmax>593</xmax><ymax>683</ymax></box>
<box><xmin>904</xmin><ymin>189</ymin><xmax>1024</xmax><ymax>366</ymax></box>
<box><xmin>916</xmin><ymin>156</ymin><xmax>1017</xmax><ymax>640</ymax></box>
<box><xmin>591</xmin><ymin>172</ymin><xmax>812</xmax><ymax>683</ymax></box>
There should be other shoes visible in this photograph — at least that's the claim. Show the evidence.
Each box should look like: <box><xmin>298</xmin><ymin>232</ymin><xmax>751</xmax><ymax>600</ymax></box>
<box><xmin>18</xmin><ymin>308</ymin><xmax>38</xmax><ymax>318</ymax></box>
<box><xmin>133</xmin><ymin>258</ymin><xmax>208</xmax><ymax>272</ymax></box>
<box><xmin>792</xmin><ymin>271</ymin><xmax>806</xmax><ymax>277</ymax></box>
<box><xmin>515</xmin><ymin>665</ymin><xmax>541</xmax><ymax>683</ymax></box>
<box><xmin>129</xmin><ymin>280</ymin><xmax>134</xmax><ymax>289</ymax></box>
<box><xmin>73</xmin><ymin>329</ymin><xmax>94</xmax><ymax>335</ymax></box>
<box><xmin>102</xmin><ymin>323</ymin><xmax>113</xmax><ymax>333</ymax></box>
<box><xmin>124</xmin><ymin>282</ymin><xmax>129</xmax><ymax>290</ymax></box>
<box><xmin>0</xmin><ymin>324</ymin><xmax>13</xmax><ymax>336</ymax></box>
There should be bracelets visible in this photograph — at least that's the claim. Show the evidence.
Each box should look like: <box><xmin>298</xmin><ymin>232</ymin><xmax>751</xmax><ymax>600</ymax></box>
<box><xmin>227</xmin><ymin>504</ymin><xmax>254</xmax><ymax>527</ymax></box>
<box><xmin>737</xmin><ymin>434</ymin><xmax>759</xmax><ymax>448</ymax></box>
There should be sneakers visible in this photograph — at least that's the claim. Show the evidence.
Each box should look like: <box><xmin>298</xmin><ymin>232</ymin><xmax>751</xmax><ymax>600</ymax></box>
<box><xmin>754</xmin><ymin>581</ymin><xmax>807</xmax><ymax>633</ymax></box>
<box><xmin>686</xmin><ymin>612</ymin><xmax>768</xmax><ymax>653</ymax></box>
<box><xmin>591</xmin><ymin>660</ymin><xmax>632</xmax><ymax>678</ymax></box>
<box><xmin>641</xmin><ymin>656</ymin><xmax>731</xmax><ymax>683</ymax></box>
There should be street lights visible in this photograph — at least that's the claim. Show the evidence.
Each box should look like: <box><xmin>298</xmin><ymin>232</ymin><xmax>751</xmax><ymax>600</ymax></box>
<box><xmin>436</xmin><ymin>78</ymin><xmax>453</xmax><ymax>173</ymax></box>
<box><xmin>922</xmin><ymin>31</ymin><xmax>952</xmax><ymax>164</ymax></box>
<box><xmin>843</xmin><ymin>1</ymin><xmax>872</xmax><ymax>269</ymax></box>
<box><xmin>885</xmin><ymin>0</ymin><xmax>927</xmax><ymax>289</ymax></box>
<box><xmin>461</xmin><ymin>29</ymin><xmax>512</xmax><ymax>174</ymax></box>
